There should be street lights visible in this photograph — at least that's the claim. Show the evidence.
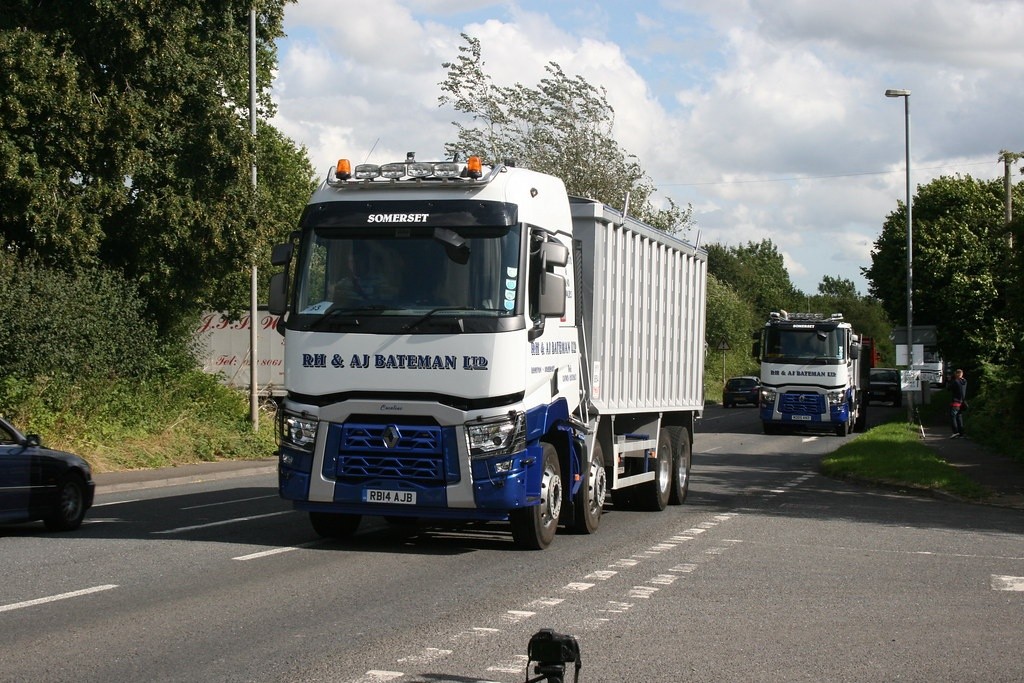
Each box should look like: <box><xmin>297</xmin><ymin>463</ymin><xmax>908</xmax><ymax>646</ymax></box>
<box><xmin>884</xmin><ymin>87</ymin><xmax>919</xmax><ymax>425</ymax></box>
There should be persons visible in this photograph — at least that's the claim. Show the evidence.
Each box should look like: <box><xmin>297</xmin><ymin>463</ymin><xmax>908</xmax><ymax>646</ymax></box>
<box><xmin>332</xmin><ymin>242</ymin><xmax>384</xmax><ymax>305</ymax></box>
<box><xmin>949</xmin><ymin>370</ymin><xmax>967</xmax><ymax>439</ymax></box>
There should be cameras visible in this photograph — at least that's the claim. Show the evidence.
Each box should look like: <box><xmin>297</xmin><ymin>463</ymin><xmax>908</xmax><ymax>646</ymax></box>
<box><xmin>528</xmin><ymin>628</ymin><xmax>576</xmax><ymax>662</ymax></box>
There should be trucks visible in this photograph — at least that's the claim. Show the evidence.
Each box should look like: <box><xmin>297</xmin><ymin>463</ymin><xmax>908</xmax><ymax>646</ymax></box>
<box><xmin>917</xmin><ymin>344</ymin><xmax>947</xmax><ymax>388</ymax></box>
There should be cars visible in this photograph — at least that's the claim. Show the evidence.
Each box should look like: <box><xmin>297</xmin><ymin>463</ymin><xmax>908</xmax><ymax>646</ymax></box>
<box><xmin>722</xmin><ymin>375</ymin><xmax>760</xmax><ymax>407</ymax></box>
<box><xmin>1</xmin><ymin>419</ymin><xmax>96</xmax><ymax>532</ymax></box>
<box><xmin>869</xmin><ymin>367</ymin><xmax>902</xmax><ymax>406</ymax></box>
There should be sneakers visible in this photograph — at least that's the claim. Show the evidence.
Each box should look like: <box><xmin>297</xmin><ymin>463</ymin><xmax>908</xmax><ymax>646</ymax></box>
<box><xmin>949</xmin><ymin>433</ymin><xmax>960</xmax><ymax>438</ymax></box>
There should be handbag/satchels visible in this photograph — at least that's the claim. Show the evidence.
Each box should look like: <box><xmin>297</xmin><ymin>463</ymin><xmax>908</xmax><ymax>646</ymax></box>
<box><xmin>960</xmin><ymin>402</ymin><xmax>967</xmax><ymax>411</ymax></box>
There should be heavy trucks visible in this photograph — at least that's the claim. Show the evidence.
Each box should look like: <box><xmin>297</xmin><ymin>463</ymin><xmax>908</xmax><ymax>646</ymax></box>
<box><xmin>760</xmin><ymin>310</ymin><xmax>875</xmax><ymax>437</ymax></box>
<box><xmin>266</xmin><ymin>150</ymin><xmax>714</xmax><ymax>548</ymax></box>
